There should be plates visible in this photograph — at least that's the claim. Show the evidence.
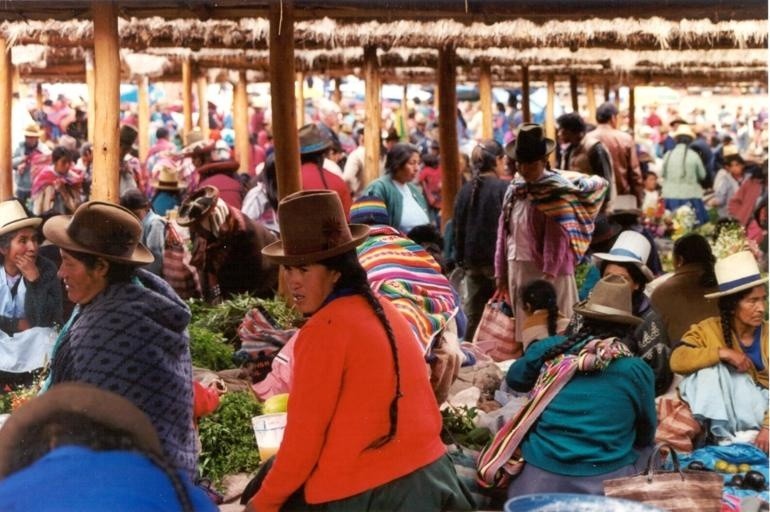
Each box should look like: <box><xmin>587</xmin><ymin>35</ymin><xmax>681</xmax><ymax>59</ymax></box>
<box><xmin>504</xmin><ymin>491</ymin><xmax>662</xmax><ymax>511</ymax></box>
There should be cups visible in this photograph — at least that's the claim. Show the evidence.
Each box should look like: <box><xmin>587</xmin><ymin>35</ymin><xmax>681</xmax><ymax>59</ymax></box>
<box><xmin>250</xmin><ymin>413</ymin><xmax>289</xmax><ymax>462</ymax></box>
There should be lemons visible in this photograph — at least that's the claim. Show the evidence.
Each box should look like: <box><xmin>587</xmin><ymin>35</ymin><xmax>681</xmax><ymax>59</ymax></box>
<box><xmin>715</xmin><ymin>460</ymin><xmax>753</xmax><ymax>474</ymax></box>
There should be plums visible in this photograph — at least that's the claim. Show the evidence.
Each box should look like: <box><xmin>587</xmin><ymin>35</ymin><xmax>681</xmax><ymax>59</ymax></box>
<box><xmin>688</xmin><ymin>461</ymin><xmax>768</xmax><ymax>492</ymax></box>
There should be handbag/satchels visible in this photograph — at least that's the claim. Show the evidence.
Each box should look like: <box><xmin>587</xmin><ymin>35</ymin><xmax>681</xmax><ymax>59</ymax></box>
<box><xmin>163</xmin><ymin>223</ymin><xmax>204</xmax><ymax>301</ymax></box>
<box><xmin>604</xmin><ymin>444</ymin><xmax>724</xmax><ymax>511</ymax></box>
<box><xmin>474</xmin><ymin>289</ymin><xmax>524</xmax><ymax>362</ymax></box>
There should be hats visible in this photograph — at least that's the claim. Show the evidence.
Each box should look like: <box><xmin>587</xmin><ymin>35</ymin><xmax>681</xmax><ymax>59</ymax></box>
<box><xmin>670</xmin><ymin>115</ymin><xmax>696</xmax><ymax>140</ymax></box>
<box><xmin>23</xmin><ymin>101</ymin><xmax>89</xmax><ymax>155</ymax></box>
<box><xmin>118</xmin><ymin>124</ymin><xmax>239</xmax><ymax>226</ymax></box>
<box><xmin>505</xmin><ymin>124</ymin><xmax>557</xmax><ymax>163</ymax></box>
<box><xmin>297</xmin><ymin>123</ymin><xmax>334</xmax><ymax>154</ymax></box>
<box><xmin>556</xmin><ymin>114</ymin><xmax>596</xmax><ymax>132</ymax></box>
<box><xmin>704</xmin><ymin>251</ymin><xmax>768</xmax><ymax>299</ymax></box>
<box><xmin>1</xmin><ymin>383</ymin><xmax>162</xmax><ymax>476</ymax></box>
<box><xmin>0</xmin><ymin>200</ymin><xmax>42</xmax><ymax>235</ymax></box>
<box><xmin>573</xmin><ymin>194</ymin><xmax>651</xmax><ymax>325</ymax></box>
<box><xmin>349</xmin><ymin>196</ymin><xmax>390</xmax><ymax>224</ymax></box>
<box><xmin>261</xmin><ymin>190</ymin><xmax>370</xmax><ymax>266</ymax></box>
<box><xmin>43</xmin><ymin>201</ymin><xmax>155</xmax><ymax>266</ymax></box>
